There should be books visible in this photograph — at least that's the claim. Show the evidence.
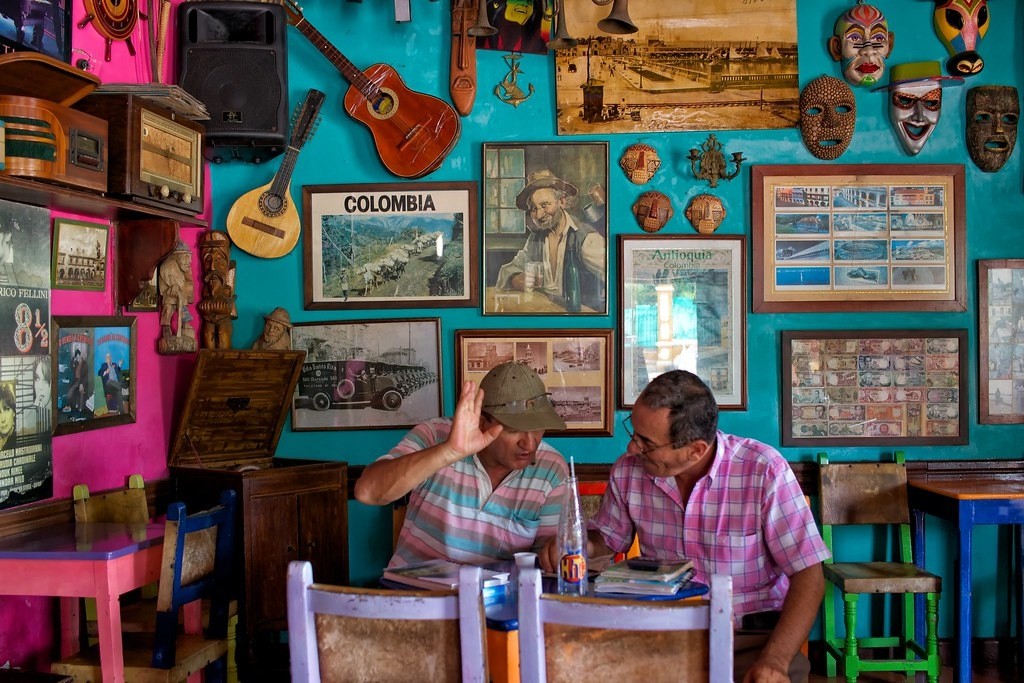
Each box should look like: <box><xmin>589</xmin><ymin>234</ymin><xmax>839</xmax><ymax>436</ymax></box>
<box><xmin>383</xmin><ymin>558</ymin><xmax>510</xmax><ymax>613</ymax></box>
<box><xmin>594</xmin><ymin>556</ymin><xmax>697</xmax><ymax>596</ymax></box>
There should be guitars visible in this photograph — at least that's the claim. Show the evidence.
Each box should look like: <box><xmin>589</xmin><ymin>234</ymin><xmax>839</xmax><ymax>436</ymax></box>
<box><xmin>264</xmin><ymin>0</ymin><xmax>460</xmax><ymax>178</ymax></box>
<box><xmin>224</xmin><ymin>87</ymin><xmax>326</xmax><ymax>261</ymax></box>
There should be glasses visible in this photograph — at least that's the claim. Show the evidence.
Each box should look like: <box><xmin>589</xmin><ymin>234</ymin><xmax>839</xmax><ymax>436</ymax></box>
<box><xmin>622</xmin><ymin>415</ymin><xmax>686</xmax><ymax>456</ymax></box>
<box><xmin>483</xmin><ymin>392</ymin><xmax>553</xmax><ymax>413</ymax></box>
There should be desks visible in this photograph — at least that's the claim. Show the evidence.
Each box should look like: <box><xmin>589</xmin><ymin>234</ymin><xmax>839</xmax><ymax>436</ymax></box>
<box><xmin>0</xmin><ymin>514</ymin><xmax>206</xmax><ymax>683</ymax></box>
<box><xmin>907</xmin><ymin>478</ymin><xmax>1024</xmax><ymax>683</ymax></box>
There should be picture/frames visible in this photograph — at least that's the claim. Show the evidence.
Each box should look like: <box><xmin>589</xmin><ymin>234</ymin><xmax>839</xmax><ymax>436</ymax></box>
<box><xmin>481</xmin><ymin>139</ymin><xmax>610</xmax><ymax>318</ymax></box>
<box><xmin>50</xmin><ymin>315</ymin><xmax>138</xmax><ymax>439</ymax></box>
<box><xmin>453</xmin><ymin>328</ymin><xmax>615</xmax><ymax>437</ymax></box>
<box><xmin>977</xmin><ymin>258</ymin><xmax>1024</xmax><ymax>426</ymax></box>
<box><xmin>615</xmin><ymin>233</ymin><xmax>748</xmax><ymax>411</ymax></box>
<box><xmin>50</xmin><ymin>215</ymin><xmax>109</xmax><ymax>291</ymax></box>
<box><xmin>301</xmin><ymin>180</ymin><xmax>480</xmax><ymax>311</ymax></box>
<box><xmin>750</xmin><ymin>164</ymin><xmax>967</xmax><ymax>313</ymax></box>
<box><xmin>286</xmin><ymin>317</ymin><xmax>445</xmax><ymax>433</ymax></box>
<box><xmin>780</xmin><ymin>329</ymin><xmax>969</xmax><ymax>447</ymax></box>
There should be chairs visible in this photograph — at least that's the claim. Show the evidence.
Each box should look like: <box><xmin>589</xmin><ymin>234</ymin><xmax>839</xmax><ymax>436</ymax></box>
<box><xmin>516</xmin><ymin>568</ymin><xmax>734</xmax><ymax>683</ymax></box>
<box><xmin>285</xmin><ymin>560</ymin><xmax>490</xmax><ymax>683</ymax></box>
<box><xmin>49</xmin><ymin>489</ymin><xmax>239</xmax><ymax>683</ymax></box>
<box><xmin>74</xmin><ymin>474</ymin><xmax>238</xmax><ymax>683</ymax></box>
<box><xmin>818</xmin><ymin>450</ymin><xmax>942</xmax><ymax>683</ymax></box>
<box><xmin>392</xmin><ymin>480</ymin><xmax>808</xmax><ymax>660</ymax></box>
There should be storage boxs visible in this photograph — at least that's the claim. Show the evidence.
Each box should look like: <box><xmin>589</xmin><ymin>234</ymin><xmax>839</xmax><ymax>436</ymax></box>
<box><xmin>0</xmin><ymin>52</ymin><xmax>207</xmax><ymax>216</ymax></box>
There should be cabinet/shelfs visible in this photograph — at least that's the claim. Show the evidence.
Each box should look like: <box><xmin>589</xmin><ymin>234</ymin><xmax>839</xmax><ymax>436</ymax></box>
<box><xmin>0</xmin><ymin>173</ymin><xmax>208</xmax><ymax>307</ymax></box>
<box><xmin>166</xmin><ymin>347</ymin><xmax>350</xmax><ymax>660</ymax></box>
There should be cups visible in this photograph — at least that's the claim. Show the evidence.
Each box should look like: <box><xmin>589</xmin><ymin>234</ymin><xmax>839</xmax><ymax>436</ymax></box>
<box><xmin>513</xmin><ymin>552</ymin><xmax>536</xmax><ymax>567</ymax></box>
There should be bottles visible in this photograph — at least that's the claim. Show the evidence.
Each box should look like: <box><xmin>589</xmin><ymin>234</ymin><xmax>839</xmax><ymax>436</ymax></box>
<box><xmin>556</xmin><ymin>476</ymin><xmax>589</xmax><ymax>597</ymax></box>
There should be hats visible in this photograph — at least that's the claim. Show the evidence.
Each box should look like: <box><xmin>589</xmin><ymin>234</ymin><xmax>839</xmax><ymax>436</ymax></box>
<box><xmin>478</xmin><ymin>362</ymin><xmax>568</xmax><ymax>432</ymax></box>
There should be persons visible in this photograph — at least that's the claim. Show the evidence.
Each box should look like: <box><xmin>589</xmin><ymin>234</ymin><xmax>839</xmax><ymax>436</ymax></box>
<box><xmin>351</xmin><ymin>358</ymin><xmax>569</xmax><ymax>592</ymax></box>
<box><xmin>198</xmin><ymin>231</ymin><xmax>237</xmax><ymax>349</ymax></box>
<box><xmin>252</xmin><ymin>307</ymin><xmax>293</xmax><ymax>351</ymax></box>
<box><xmin>557</xmin><ymin>63</ymin><xmax>627</xmax><ymax>120</ymax></box>
<box><xmin>33</xmin><ymin>361</ymin><xmax>52</xmax><ymax>410</ymax></box>
<box><xmin>0</xmin><ymin>383</ymin><xmax>16</xmax><ymax>450</ymax></box>
<box><xmin>159</xmin><ymin>242</ymin><xmax>194</xmax><ymax>337</ymax></box>
<box><xmin>540</xmin><ymin>370</ymin><xmax>833</xmax><ymax>683</ymax></box>
